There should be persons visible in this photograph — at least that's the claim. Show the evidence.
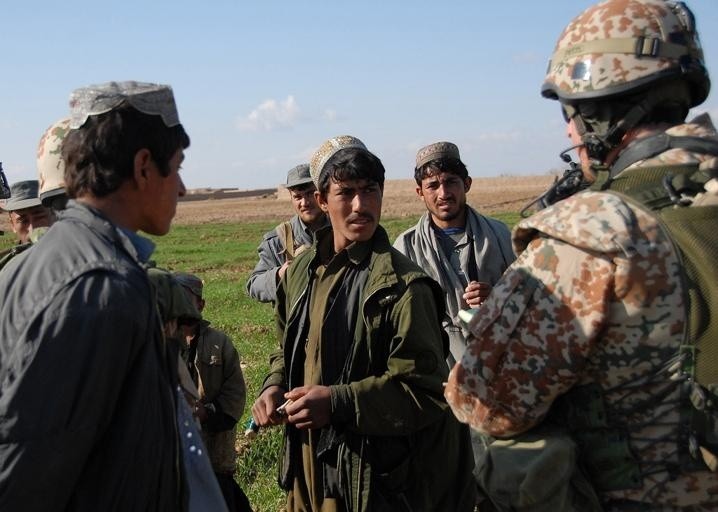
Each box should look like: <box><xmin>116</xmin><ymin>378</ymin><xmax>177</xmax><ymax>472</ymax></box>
<box><xmin>249</xmin><ymin>134</ymin><xmax>447</xmax><ymax>512</ymax></box>
<box><xmin>173</xmin><ymin>272</ymin><xmax>245</xmax><ymax>511</ymax></box>
<box><xmin>447</xmin><ymin>0</ymin><xmax>718</xmax><ymax>512</ymax></box>
<box><xmin>394</xmin><ymin>142</ymin><xmax>516</xmax><ymax>334</ymax></box>
<box><xmin>248</xmin><ymin>164</ymin><xmax>334</xmax><ymax>301</ymax></box>
<box><xmin>37</xmin><ymin>116</ymin><xmax>253</xmax><ymax>512</ymax></box>
<box><xmin>0</xmin><ymin>181</ymin><xmax>53</xmax><ymax>270</ymax></box>
<box><xmin>0</xmin><ymin>80</ymin><xmax>190</xmax><ymax>512</ymax></box>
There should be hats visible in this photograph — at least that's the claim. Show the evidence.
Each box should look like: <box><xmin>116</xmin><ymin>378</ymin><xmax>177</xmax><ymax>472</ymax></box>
<box><xmin>36</xmin><ymin>118</ymin><xmax>70</xmax><ymax>208</ymax></box>
<box><xmin>172</xmin><ymin>269</ymin><xmax>203</xmax><ymax>298</ymax></box>
<box><xmin>67</xmin><ymin>80</ymin><xmax>182</xmax><ymax>130</ymax></box>
<box><xmin>284</xmin><ymin>162</ymin><xmax>313</xmax><ymax>188</ymax></box>
<box><xmin>309</xmin><ymin>135</ymin><xmax>368</xmax><ymax>192</ymax></box>
<box><xmin>1</xmin><ymin>179</ymin><xmax>42</xmax><ymax>212</ymax></box>
<box><xmin>415</xmin><ymin>141</ymin><xmax>460</xmax><ymax>170</ymax></box>
<box><xmin>541</xmin><ymin>0</ymin><xmax>712</xmax><ymax>109</ymax></box>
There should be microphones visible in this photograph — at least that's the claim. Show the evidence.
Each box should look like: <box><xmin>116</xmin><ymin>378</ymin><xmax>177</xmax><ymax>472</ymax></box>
<box><xmin>559</xmin><ymin>145</ymin><xmax>582</xmax><ymax>163</ymax></box>
